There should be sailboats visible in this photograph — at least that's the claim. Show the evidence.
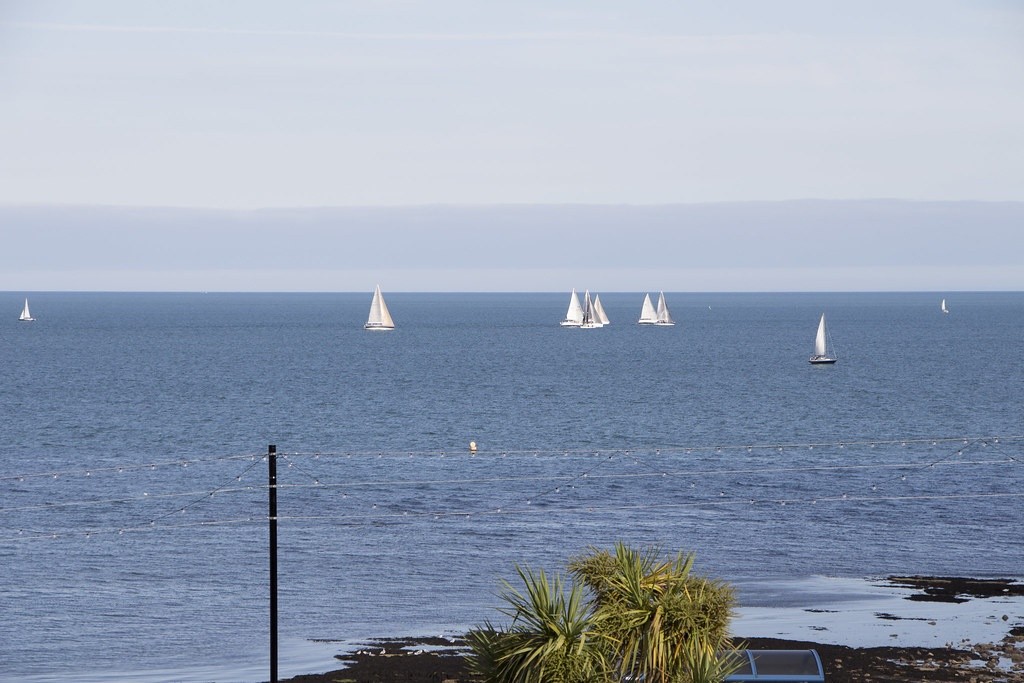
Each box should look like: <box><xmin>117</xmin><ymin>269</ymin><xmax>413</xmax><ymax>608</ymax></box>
<box><xmin>808</xmin><ymin>312</ymin><xmax>839</xmax><ymax>364</ymax></box>
<box><xmin>559</xmin><ymin>287</ymin><xmax>585</xmax><ymax>326</ymax></box>
<box><xmin>653</xmin><ymin>287</ymin><xmax>676</xmax><ymax>326</ymax></box>
<box><xmin>592</xmin><ymin>293</ymin><xmax>610</xmax><ymax>325</ymax></box>
<box><xmin>18</xmin><ymin>297</ymin><xmax>37</xmax><ymax>321</ymax></box>
<box><xmin>579</xmin><ymin>289</ymin><xmax>604</xmax><ymax>329</ymax></box>
<box><xmin>638</xmin><ymin>292</ymin><xmax>657</xmax><ymax>325</ymax></box>
<box><xmin>363</xmin><ymin>284</ymin><xmax>395</xmax><ymax>331</ymax></box>
